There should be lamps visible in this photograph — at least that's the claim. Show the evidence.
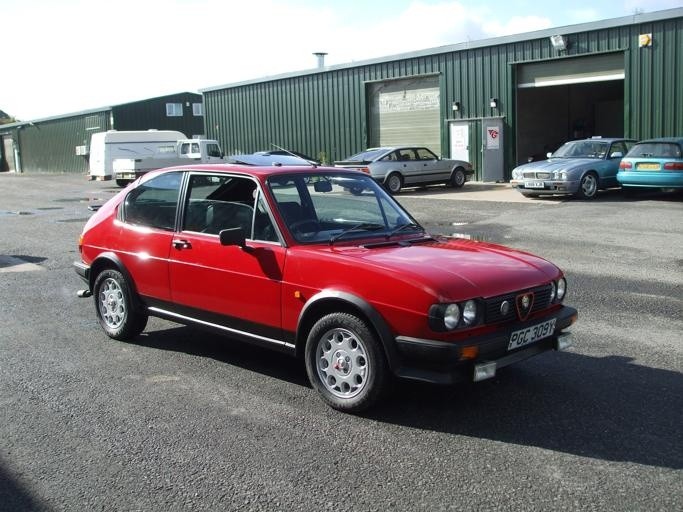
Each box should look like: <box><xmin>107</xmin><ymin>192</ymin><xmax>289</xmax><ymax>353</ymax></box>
<box><xmin>550</xmin><ymin>35</ymin><xmax>567</xmax><ymax>50</ymax></box>
<box><xmin>452</xmin><ymin>101</ymin><xmax>459</xmax><ymax>111</ymax></box>
<box><xmin>490</xmin><ymin>98</ymin><xmax>496</xmax><ymax>108</ymax></box>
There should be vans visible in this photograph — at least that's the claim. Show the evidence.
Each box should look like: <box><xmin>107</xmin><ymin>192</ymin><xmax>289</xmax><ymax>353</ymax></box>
<box><xmin>86</xmin><ymin>128</ymin><xmax>188</xmax><ymax>182</ymax></box>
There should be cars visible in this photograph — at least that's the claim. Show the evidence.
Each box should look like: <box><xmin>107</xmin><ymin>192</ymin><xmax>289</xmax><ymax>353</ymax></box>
<box><xmin>509</xmin><ymin>135</ymin><xmax>640</xmax><ymax>198</ymax></box>
<box><xmin>257</xmin><ymin>149</ymin><xmax>321</xmax><ymax>183</ymax></box>
<box><xmin>74</xmin><ymin>163</ymin><xmax>578</xmax><ymax>411</ymax></box>
<box><xmin>336</xmin><ymin>145</ymin><xmax>474</xmax><ymax>193</ymax></box>
<box><xmin>616</xmin><ymin>137</ymin><xmax>683</xmax><ymax>194</ymax></box>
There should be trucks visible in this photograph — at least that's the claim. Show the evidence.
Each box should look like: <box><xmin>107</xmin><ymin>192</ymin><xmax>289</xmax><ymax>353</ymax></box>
<box><xmin>113</xmin><ymin>139</ymin><xmax>226</xmax><ymax>181</ymax></box>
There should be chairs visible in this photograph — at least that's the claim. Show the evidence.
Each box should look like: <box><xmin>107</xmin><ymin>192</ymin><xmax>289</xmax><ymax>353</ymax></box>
<box><xmin>403</xmin><ymin>154</ymin><xmax>410</xmax><ymax>160</ymax></box>
<box><xmin>207</xmin><ymin>201</ymin><xmax>262</xmax><ymax>239</ymax></box>
<box><xmin>278</xmin><ymin>200</ymin><xmax>318</xmax><ymax>232</ymax></box>
